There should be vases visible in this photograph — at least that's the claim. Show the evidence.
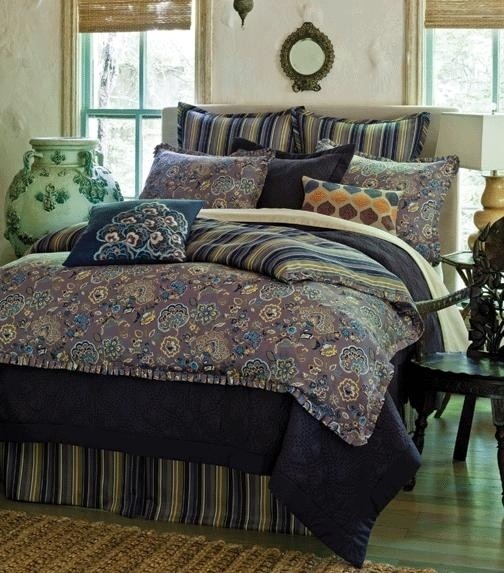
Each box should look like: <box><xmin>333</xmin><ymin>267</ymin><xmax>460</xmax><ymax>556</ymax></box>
<box><xmin>4</xmin><ymin>138</ymin><xmax>124</xmax><ymax>259</ymax></box>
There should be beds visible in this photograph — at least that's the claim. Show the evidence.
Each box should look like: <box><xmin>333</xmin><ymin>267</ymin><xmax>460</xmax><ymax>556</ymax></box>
<box><xmin>0</xmin><ymin>104</ymin><xmax>464</xmax><ymax>568</ymax></box>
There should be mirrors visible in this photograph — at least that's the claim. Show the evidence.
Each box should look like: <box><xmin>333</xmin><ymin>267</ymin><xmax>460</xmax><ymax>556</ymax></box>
<box><xmin>280</xmin><ymin>21</ymin><xmax>334</xmax><ymax>93</ymax></box>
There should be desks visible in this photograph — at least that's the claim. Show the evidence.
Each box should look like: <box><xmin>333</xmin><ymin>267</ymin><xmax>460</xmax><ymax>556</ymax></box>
<box><xmin>440</xmin><ymin>249</ymin><xmax>504</xmax><ymax>311</ymax></box>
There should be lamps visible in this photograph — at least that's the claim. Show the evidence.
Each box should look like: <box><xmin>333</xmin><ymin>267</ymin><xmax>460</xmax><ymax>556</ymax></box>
<box><xmin>433</xmin><ymin>112</ymin><xmax>504</xmax><ymax>261</ymax></box>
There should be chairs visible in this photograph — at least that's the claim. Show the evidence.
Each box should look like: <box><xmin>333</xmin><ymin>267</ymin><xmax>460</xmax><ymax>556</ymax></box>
<box><xmin>400</xmin><ymin>216</ymin><xmax>504</xmax><ymax>505</ymax></box>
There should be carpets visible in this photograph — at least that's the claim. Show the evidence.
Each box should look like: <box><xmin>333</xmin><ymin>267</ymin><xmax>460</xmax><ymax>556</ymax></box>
<box><xmin>0</xmin><ymin>509</ymin><xmax>441</xmax><ymax>573</ymax></box>
